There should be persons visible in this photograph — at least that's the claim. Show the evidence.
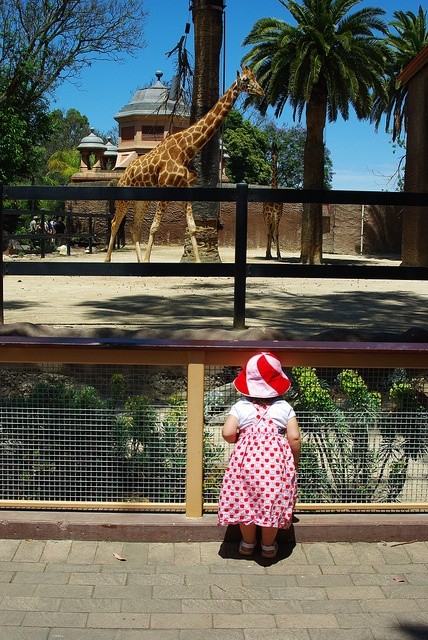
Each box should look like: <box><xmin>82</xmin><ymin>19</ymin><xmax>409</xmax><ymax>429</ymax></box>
<box><xmin>28</xmin><ymin>214</ymin><xmax>83</xmax><ymax>247</ymax></box>
<box><xmin>216</xmin><ymin>351</ymin><xmax>302</xmax><ymax>559</ymax></box>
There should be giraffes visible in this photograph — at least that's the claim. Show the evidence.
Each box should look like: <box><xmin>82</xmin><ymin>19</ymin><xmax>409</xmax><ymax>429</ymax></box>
<box><xmin>264</xmin><ymin>141</ymin><xmax>287</xmax><ymax>261</ymax></box>
<box><xmin>104</xmin><ymin>64</ymin><xmax>266</xmax><ymax>263</ymax></box>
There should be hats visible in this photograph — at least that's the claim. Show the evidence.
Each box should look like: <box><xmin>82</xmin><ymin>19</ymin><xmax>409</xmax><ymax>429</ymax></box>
<box><xmin>233</xmin><ymin>352</ymin><xmax>291</xmax><ymax>399</ymax></box>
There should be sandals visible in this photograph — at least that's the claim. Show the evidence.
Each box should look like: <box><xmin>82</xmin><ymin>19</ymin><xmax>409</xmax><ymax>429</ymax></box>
<box><xmin>239</xmin><ymin>540</ymin><xmax>258</xmax><ymax>555</ymax></box>
<box><xmin>261</xmin><ymin>541</ymin><xmax>278</xmax><ymax>557</ymax></box>
